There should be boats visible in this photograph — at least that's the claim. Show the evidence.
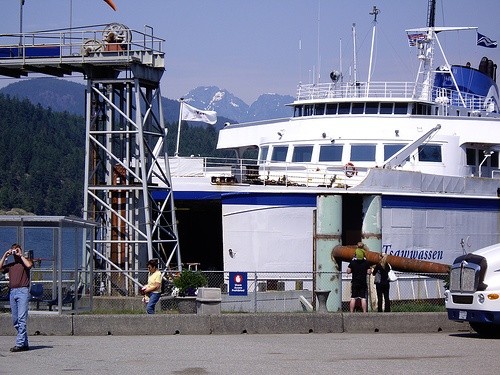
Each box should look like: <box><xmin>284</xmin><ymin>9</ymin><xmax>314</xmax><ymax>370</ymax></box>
<box><xmin>120</xmin><ymin>0</ymin><xmax>500</xmax><ymax>300</ymax></box>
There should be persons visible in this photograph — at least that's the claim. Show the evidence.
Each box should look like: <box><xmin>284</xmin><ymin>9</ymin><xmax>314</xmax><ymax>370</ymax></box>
<box><xmin>0</xmin><ymin>243</ymin><xmax>32</xmax><ymax>352</ymax></box>
<box><xmin>347</xmin><ymin>242</ymin><xmax>372</xmax><ymax>312</ymax></box>
<box><xmin>142</xmin><ymin>260</ymin><xmax>162</xmax><ymax>314</ymax></box>
<box><xmin>372</xmin><ymin>254</ymin><xmax>390</xmax><ymax>312</ymax></box>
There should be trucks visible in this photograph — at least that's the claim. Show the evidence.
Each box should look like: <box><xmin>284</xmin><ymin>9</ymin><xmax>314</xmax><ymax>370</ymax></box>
<box><xmin>445</xmin><ymin>242</ymin><xmax>500</xmax><ymax>338</ymax></box>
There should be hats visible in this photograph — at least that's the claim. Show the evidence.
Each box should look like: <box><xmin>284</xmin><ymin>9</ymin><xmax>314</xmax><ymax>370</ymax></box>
<box><xmin>358</xmin><ymin>242</ymin><xmax>364</xmax><ymax>246</ymax></box>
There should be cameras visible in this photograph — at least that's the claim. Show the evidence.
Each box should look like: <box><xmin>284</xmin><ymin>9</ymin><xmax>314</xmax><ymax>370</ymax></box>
<box><xmin>12</xmin><ymin>250</ymin><xmax>17</xmax><ymax>254</ymax></box>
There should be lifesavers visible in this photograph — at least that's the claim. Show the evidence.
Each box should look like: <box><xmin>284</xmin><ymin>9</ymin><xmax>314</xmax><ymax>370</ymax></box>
<box><xmin>344</xmin><ymin>163</ymin><xmax>355</xmax><ymax>178</ymax></box>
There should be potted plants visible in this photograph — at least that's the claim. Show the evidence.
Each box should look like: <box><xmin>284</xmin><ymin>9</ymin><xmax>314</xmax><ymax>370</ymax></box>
<box><xmin>171</xmin><ymin>270</ymin><xmax>208</xmax><ymax>314</ymax></box>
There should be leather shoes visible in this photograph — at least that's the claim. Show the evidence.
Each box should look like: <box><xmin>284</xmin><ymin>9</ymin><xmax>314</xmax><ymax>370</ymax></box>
<box><xmin>10</xmin><ymin>346</ymin><xmax>30</xmax><ymax>352</ymax></box>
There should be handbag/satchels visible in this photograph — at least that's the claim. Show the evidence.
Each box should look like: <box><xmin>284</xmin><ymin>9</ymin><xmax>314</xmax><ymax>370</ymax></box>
<box><xmin>374</xmin><ymin>270</ymin><xmax>380</xmax><ymax>283</ymax></box>
<box><xmin>387</xmin><ymin>263</ymin><xmax>398</xmax><ymax>282</ymax></box>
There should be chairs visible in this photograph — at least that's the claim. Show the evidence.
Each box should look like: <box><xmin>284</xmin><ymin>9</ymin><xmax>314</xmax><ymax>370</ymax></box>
<box><xmin>0</xmin><ymin>284</ymin><xmax>85</xmax><ymax>311</ymax></box>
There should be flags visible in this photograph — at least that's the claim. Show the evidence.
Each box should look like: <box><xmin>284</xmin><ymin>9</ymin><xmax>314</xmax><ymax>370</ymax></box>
<box><xmin>407</xmin><ymin>33</ymin><xmax>423</xmax><ymax>47</ymax></box>
<box><xmin>179</xmin><ymin>102</ymin><xmax>217</xmax><ymax>125</ymax></box>
<box><xmin>477</xmin><ymin>32</ymin><xmax>497</xmax><ymax>48</ymax></box>
